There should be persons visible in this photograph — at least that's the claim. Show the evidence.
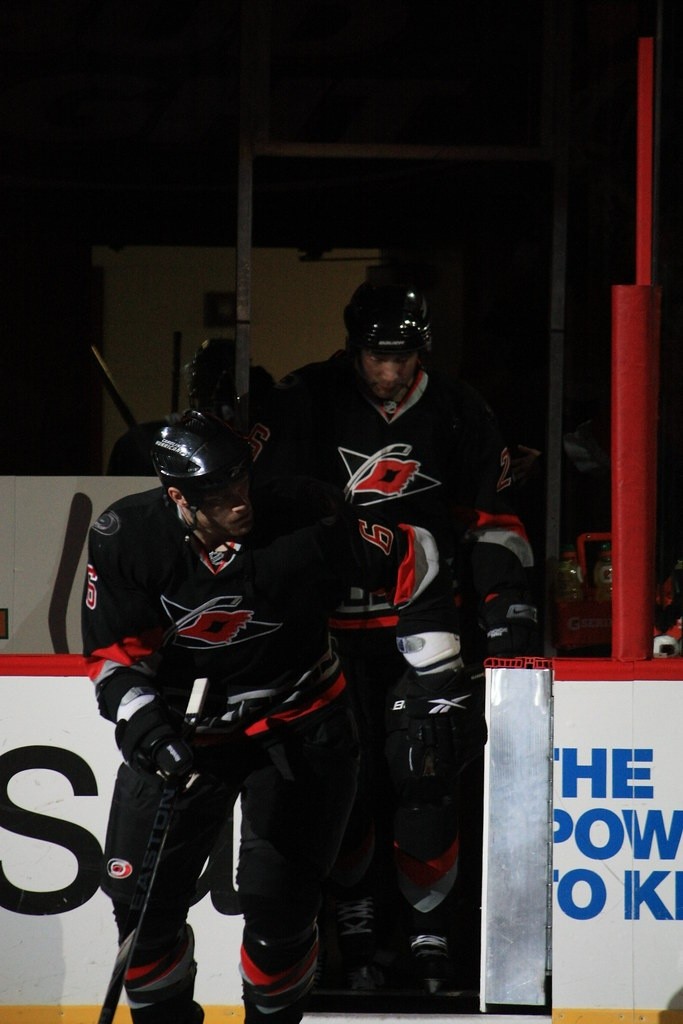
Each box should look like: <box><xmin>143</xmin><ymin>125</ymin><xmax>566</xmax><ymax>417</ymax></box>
<box><xmin>509</xmin><ymin>438</ymin><xmax>609</xmax><ymax>517</ymax></box>
<box><xmin>80</xmin><ymin>411</ymin><xmax>332</xmax><ymax>1023</ymax></box>
<box><xmin>187</xmin><ymin>291</ymin><xmax>275</xmax><ymax>410</ymax></box>
<box><xmin>180</xmin><ymin>275</ymin><xmax>545</xmax><ymax>977</ymax></box>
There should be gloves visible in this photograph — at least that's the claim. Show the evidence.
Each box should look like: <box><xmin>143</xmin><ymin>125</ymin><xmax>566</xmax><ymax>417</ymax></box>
<box><xmin>143</xmin><ymin>735</ymin><xmax>221</xmax><ymax>812</ymax></box>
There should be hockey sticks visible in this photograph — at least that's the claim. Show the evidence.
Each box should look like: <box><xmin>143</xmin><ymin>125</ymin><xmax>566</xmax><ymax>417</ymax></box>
<box><xmin>91</xmin><ymin>675</ymin><xmax>211</xmax><ymax>1024</ymax></box>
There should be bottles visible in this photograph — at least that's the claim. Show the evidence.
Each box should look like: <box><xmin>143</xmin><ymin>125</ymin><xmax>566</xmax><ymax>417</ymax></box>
<box><xmin>558</xmin><ymin>536</ymin><xmax>584</xmax><ymax>601</ymax></box>
<box><xmin>594</xmin><ymin>543</ymin><xmax>612</xmax><ymax>601</ymax></box>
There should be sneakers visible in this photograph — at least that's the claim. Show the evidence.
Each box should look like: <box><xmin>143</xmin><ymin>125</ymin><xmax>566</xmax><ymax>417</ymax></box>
<box><xmin>408</xmin><ymin>930</ymin><xmax>449</xmax><ymax>995</ymax></box>
<box><xmin>335</xmin><ymin>899</ymin><xmax>381</xmax><ymax>993</ymax></box>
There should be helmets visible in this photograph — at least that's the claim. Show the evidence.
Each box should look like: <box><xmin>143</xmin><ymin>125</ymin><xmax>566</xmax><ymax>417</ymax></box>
<box><xmin>150</xmin><ymin>409</ymin><xmax>254</xmax><ymax>504</ymax></box>
<box><xmin>344</xmin><ymin>278</ymin><xmax>431</xmax><ymax>354</ymax></box>
